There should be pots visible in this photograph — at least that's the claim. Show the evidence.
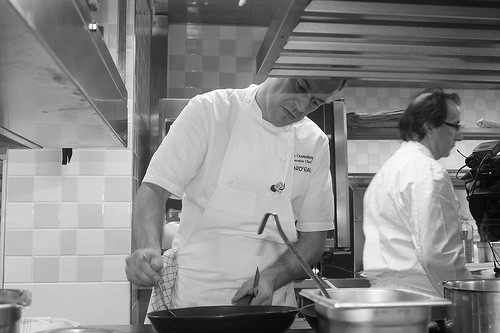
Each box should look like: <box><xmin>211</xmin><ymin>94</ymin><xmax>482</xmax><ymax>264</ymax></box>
<box><xmin>0</xmin><ymin>288</ymin><xmax>32</xmax><ymax>333</ymax></box>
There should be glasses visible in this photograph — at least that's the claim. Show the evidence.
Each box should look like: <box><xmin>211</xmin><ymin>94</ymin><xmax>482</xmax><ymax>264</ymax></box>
<box><xmin>439</xmin><ymin>121</ymin><xmax>462</xmax><ymax>134</ymax></box>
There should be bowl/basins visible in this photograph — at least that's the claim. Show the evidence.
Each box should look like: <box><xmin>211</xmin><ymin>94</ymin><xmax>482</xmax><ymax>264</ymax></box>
<box><xmin>300</xmin><ymin>303</ymin><xmax>319</xmax><ymax>333</ymax></box>
<box><xmin>146</xmin><ymin>305</ymin><xmax>300</xmax><ymax>333</ymax></box>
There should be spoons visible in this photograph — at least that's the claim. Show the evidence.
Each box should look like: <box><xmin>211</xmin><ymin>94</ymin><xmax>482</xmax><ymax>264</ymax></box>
<box><xmin>142</xmin><ymin>252</ymin><xmax>176</xmax><ymax>316</ymax></box>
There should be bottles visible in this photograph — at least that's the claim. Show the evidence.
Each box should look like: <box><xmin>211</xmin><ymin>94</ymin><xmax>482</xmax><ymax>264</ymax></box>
<box><xmin>461</xmin><ymin>218</ymin><xmax>474</xmax><ymax>263</ymax></box>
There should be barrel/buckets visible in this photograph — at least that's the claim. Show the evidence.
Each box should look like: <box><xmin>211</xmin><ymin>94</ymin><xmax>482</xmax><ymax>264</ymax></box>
<box><xmin>438</xmin><ymin>278</ymin><xmax>500</xmax><ymax>333</ymax></box>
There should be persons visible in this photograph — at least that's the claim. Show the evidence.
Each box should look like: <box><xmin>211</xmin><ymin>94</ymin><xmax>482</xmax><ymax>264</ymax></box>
<box><xmin>125</xmin><ymin>75</ymin><xmax>347</xmax><ymax>325</ymax></box>
<box><xmin>362</xmin><ymin>87</ymin><xmax>475</xmax><ymax>299</ymax></box>
